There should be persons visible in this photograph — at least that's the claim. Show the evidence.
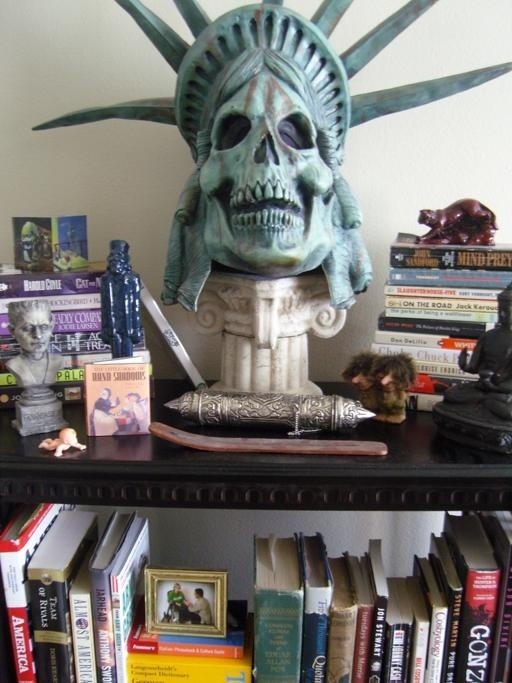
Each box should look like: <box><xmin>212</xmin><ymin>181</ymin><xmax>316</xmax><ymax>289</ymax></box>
<box><xmin>98</xmin><ymin>237</ymin><xmax>143</xmax><ymax>366</ymax></box>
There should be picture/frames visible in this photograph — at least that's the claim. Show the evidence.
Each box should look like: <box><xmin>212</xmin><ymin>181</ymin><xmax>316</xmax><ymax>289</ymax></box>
<box><xmin>141</xmin><ymin>566</ymin><xmax>229</xmax><ymax>638</ymax></box>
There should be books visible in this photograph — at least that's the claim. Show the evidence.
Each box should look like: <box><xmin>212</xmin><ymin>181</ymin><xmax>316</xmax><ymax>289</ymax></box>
<box><xmin>0</xmin><ymin>260</ymin><xmax>151</xmax><ymax>452</ymax></box>
<box><xmin>369</xmin><ymin>229</ymin><xmax>512</xmax><ymax>422</ymax></box>
